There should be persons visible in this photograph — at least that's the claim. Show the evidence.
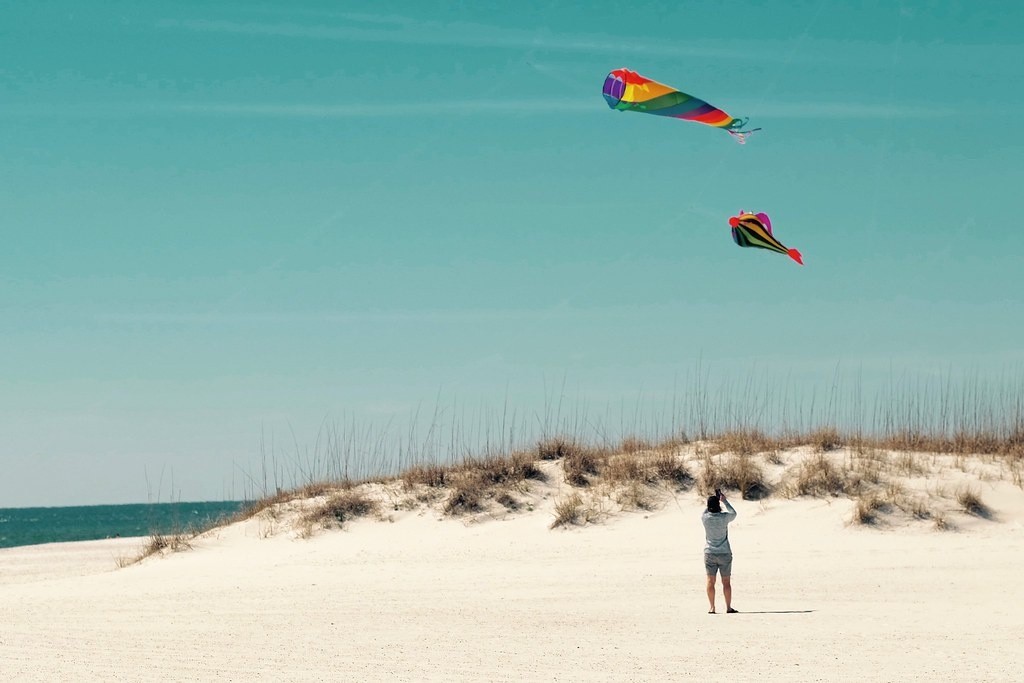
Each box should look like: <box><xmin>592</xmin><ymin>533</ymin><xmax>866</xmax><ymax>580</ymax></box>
<box><xmin>702</xmin><ymin>493</ymin><xmax>738</xmax><ymax>614</ymax></box>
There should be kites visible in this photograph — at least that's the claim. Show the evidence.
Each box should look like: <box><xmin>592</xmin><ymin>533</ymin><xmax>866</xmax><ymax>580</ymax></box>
<box><xmin>728</xmin><ymin>209</ymin><xmax>804</xmax><ymax>265</ymax></box>
<box><xmin>602</xmin><ymin>68</ymin><xmax>762</xmax><ymax>145</ymax></box>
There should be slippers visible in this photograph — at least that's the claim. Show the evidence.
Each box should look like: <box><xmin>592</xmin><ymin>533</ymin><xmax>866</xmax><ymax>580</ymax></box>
<box><xmin>708</xmin><ymin>610</ymin><xmax>715</xmax><ymax>613</ymax></box>
<box><xmin>727</xmin><ymin>608</ymin><xmax>738</xmax><ymax>613</ymax></box>
